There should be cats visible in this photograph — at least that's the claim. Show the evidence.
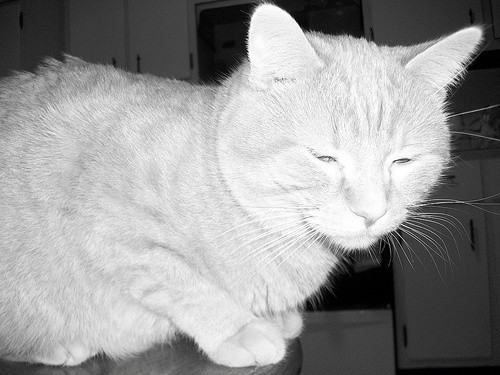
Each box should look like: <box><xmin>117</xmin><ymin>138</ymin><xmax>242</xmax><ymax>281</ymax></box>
<box><xmin>0</xmin><ymin>2</ymin><xmax>484</xmax><ymax>368</ymax></box>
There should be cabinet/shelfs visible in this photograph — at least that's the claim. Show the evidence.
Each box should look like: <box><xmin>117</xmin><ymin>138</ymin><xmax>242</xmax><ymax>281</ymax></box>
<box><xmin>0</xmin><ymin>0</ymin><xmax>201</xmax><ymax>87</ymax></box>
<box><xmin>365</xmin><ymin>0</ymin><xmax>500</xmax><ymax>369</ymax></box>
